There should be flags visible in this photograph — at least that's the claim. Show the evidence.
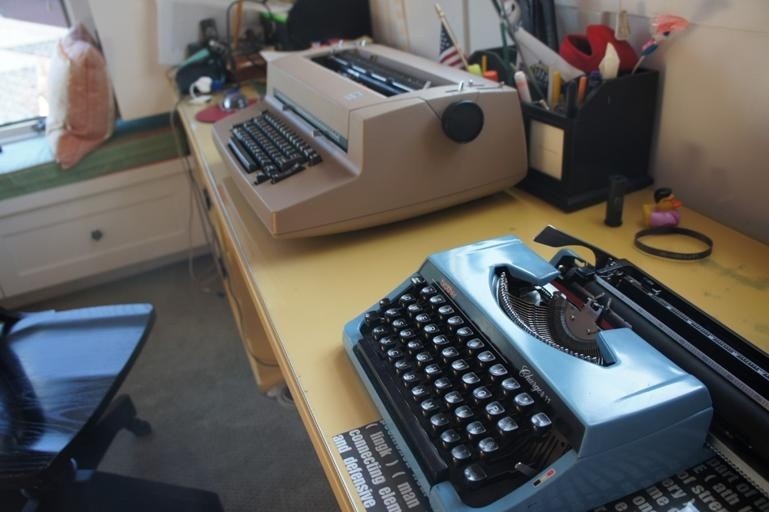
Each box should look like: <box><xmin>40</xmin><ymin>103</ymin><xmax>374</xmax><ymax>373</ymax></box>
<box><xmin>439</xmin><ymin>22</ymin><xmax>464</xmax><ymax>71</ymax></box>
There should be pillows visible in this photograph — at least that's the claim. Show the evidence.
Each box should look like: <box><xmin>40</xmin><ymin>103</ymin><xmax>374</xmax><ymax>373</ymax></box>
<box><xmin>43</xmin><ymin>23</ymin><xmax>124</xmax><ymax>177</ymax></box>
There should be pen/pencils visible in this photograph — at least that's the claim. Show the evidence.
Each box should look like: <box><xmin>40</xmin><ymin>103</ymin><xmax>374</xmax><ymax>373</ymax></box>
<box><xmin>467</xmin><ymin>64</ymin><xmax>588</xmax><ymax>111</ymax></box>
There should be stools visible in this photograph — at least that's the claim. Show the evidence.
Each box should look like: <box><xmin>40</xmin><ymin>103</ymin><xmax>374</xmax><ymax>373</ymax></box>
<box><xmin>0</xmin><ymin>302</ymin><xmax>223</xmax><ymax>512</ymax></box>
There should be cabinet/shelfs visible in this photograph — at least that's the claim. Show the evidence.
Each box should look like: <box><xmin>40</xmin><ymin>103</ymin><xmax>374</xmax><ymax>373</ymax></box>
<box><xmin>0</xmin><ymin>155</ymin><xmax>211</xmax><ymax>304</ymax></box>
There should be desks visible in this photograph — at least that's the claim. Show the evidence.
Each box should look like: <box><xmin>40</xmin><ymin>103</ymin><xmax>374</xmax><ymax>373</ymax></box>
<box><xmin>173</xmin><ymin>78</ymin><xmax>766</xmax><ymax>512</ymax></box>
